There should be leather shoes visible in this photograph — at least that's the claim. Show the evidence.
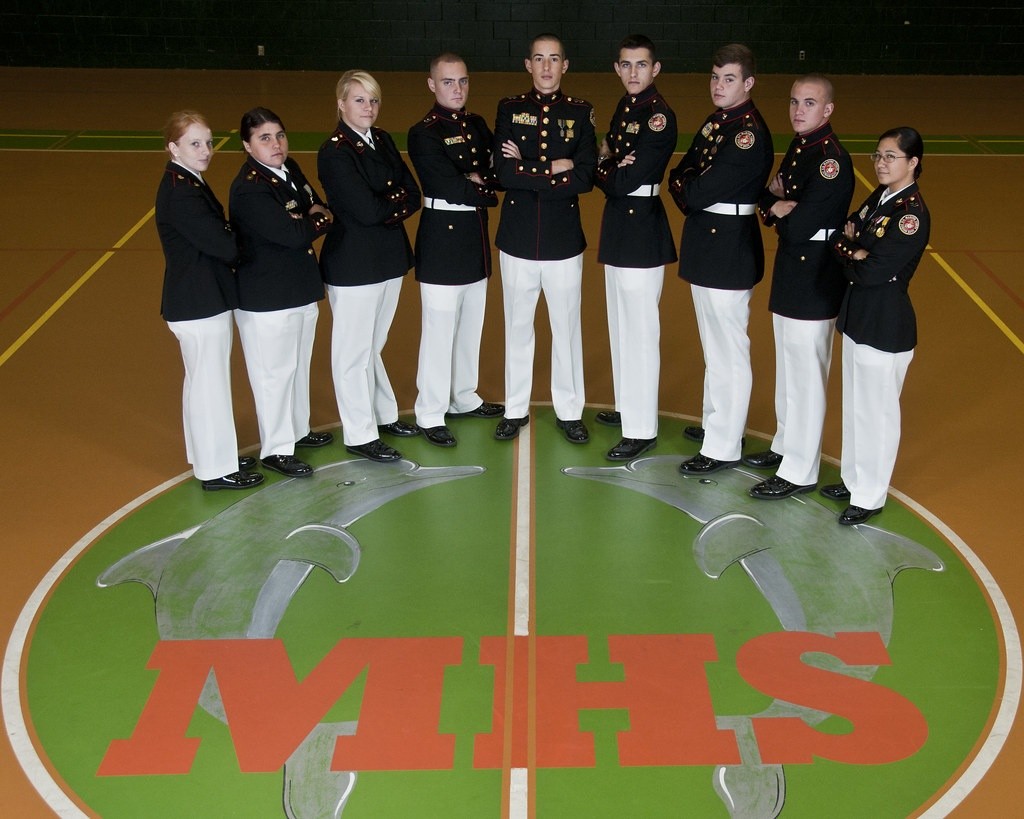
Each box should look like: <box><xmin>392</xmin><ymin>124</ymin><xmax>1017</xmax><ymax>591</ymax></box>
<box><xmin>742</xmin><ymin>449</ymin><xmax>783</xmax><ymax>469</ymax></box>
<box><xmin>751</xmin><ymin>473</ymin><xmax>817</xmax><ymax>500</ymax></box>
<box><xmin>445</xmin><ymin>403</ymin><xmax>505</xmax><ymax>418</ymax></box>
<box><xmin>680</xmin><ymin>452</ymin><xmax>742</xmax><ymax>475</ymax></box>
<box><xmin>261</xmin><ymin>455</ymin><xmax>313</xmax><ymax>478</ymax></box>
<box><xmin>839</xmin><ymin>503</ymin><xmax>882</xmax><ymax>525</ymax></box>
<box><xmin>596</xmin><ymin>411</ymin><xmax>621</xmax><ymax>426</ymax></box>
<box><xmin>495</xmin><ymin>414</ymin><xmax>529</xmax><ymax>439</ymax></box>
<box><xmin>607</xmin><ymin>436</ymin><xmax>657</xmax><ymax>460</ymax></box>
<box><xmin>683</xmin><ymin>425</ymin><xmax>745</xmax><ymax>451</ymax></box>
<box><xmin>378</xmin><ymin>419</ymin><xmax>421</xmax><ymax>437</ymax></box>
<box><xmin>556</xmin><ymin>418</ymin><xmax>590</xmax><ymax>443</ymax></box>
<box><xmin>238</xmin><ymin>456</ymin><xmax>257</xmax><ymax>470</ymax></box>
<box><xmin>419</xmin><ymin>426</ymin><xmax>457</xmax><ymax>447</ymax></box>
<box><xmin>295</xmin><ymin>430</ymin><xmax>333</xmax><ymax>447</ymax></box>
<box><xmin>346</xmin><ymin>439</ymin><xmax>402</xmax><ymax>463</ymax></box>
<box><xmin>820</xmin><ymin>481</ymin><xmax>851</xmax><ymax>500</ymax></box>
<box><xmin>202</xmin><ymin>470</ymin><xmax>265</xmax><ymax>491</ymax></box>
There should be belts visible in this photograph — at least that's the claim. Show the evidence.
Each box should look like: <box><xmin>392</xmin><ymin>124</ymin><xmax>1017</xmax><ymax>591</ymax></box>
<box><xmin>424</xmin><ymin>197</ymin><xmax>478</xmax><ymax>211</ymax></box>
<box><xmin>703</xmin><ymin>202</ymin><xmax>758</xmax><ymax>216</ymax></box>
<box><xmin>628</xmin><ymin>184</ymin><xmax>662</xmax><ymax>197</ymax></box>
<box><xmin>808</xmin><ymin>228</ymin><xmax>837</xmax><ymax>243</ymax></box>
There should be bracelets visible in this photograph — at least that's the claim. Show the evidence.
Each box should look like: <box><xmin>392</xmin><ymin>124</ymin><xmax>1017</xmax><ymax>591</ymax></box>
<box><xmin>597</xmin><ymin>153</ymin><xmax>610</xmax><ymax>160</ymax></box>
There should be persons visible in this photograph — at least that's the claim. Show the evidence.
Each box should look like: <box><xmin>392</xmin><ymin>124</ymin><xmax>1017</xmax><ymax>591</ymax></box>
<box><xmin>740</xmin><ymin>74</ymin><xmax>855</xmax><ymax>503</ymax></box>
<box><xmin>407</xmin><ymin>53</ymin><xmax>507</xmax><ymax>449</ymax></box>
<box><xmin>492</xmin><ymin>33</ymin><xmax>600</xmax><ymax>446</ymax></box>
<box><xmin>318</xmin><ymin>70</ymin><xmax>420</xmax><ymax>464</ymax></box>
<box><xmin>592</xmin><ymin>33</ymin><xmax>679</xmax><ymax>465</ymax></box>
<box><xmin>667</xmin><ymin>44</ymin><xmax>774</xmax><ymax>477</ymax></box>
<box><xmin>827</xmin><ymin>127</ymin><xmax>931</xmax><ymax>526</ymax></box>
<box><xmin>227</xmin><ymin>107</ymin><xmax>333</xmax><ymax>479</ymax></box>
<box><xmin>154</xmin><ymin>108</ymin><xmax>264</xmax><ymax>493</ymax></box>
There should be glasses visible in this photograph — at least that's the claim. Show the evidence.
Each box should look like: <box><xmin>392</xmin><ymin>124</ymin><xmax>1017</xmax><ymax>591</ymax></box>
<box><xmin>871</xmin><ymin>154</ymin><xmax>916</xmax><ymax>163</ymax></box>
